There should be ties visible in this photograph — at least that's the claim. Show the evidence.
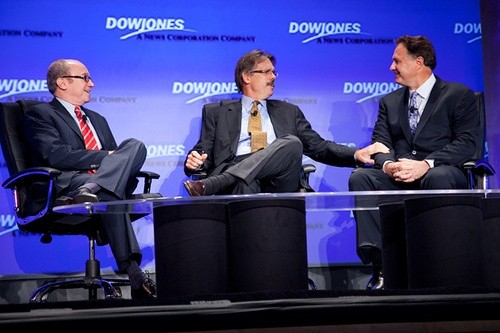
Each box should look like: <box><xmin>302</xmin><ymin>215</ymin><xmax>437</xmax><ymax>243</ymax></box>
<box><xmin>248</xmin><ymin>100</ymin><xmax>267</xmax><ymax>153</ymax></box>
<box><xmin>408</xmin><ymin>91</ymin><xmax>421</xmax><ymax>138</ymax></box>
<box><xmin>74</xmin><ymin>107</ymin><xmax>100</xmax><ymax>175</ymax></box>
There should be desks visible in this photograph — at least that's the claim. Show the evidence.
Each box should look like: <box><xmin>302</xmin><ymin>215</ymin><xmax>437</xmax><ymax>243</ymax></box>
<box><xmin>51</xmin><ymin>188</ymin><xmax>500</xmax><ymax>306</ymax></box>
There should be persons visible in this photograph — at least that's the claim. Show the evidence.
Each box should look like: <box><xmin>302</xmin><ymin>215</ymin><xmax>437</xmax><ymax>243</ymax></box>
<box><xmin>26</xmin><ymin>59</ymin><xmax>157</xmax><ymax>301</ymax></box>
<box><xmin>348</xmin><ymin>35</ymin><xmax>483</xmax><ymax>290</ymax></box>
<box><xmin>183</xmin><ymin>50</ymin><xmax>390</xmax><ymax>197</ymax></box>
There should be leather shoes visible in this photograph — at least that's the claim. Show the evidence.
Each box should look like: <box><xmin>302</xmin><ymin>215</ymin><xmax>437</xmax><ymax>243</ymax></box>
<box><xmin>183</xmin><ymin>178</ymin><xmax>205</xmax><ymax>197</ymax></box>
<box><xmin>367</xmin><ymin>264</ymin><xmax>385</xmax><ymax>290</ymax></box>
<box><xmin>54</xmin><ymin>188</ymin><xmax>99</xmax><ymax>205</ymax></box>
<box><xmin>131</xmin><ymin>270</ymin><xmax>157</xmax><ymax>299</ymax></box>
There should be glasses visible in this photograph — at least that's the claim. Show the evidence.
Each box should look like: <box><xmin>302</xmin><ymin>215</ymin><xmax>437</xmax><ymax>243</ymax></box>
<box><xmin>246</xmin><ymin>70</ymin><xmax>279</xmax><ymax>77</ymax></box>
<box><xmin>56</xmin><ymin>75</ymin><xmax>91</xmax><ymax>82</ymax></box>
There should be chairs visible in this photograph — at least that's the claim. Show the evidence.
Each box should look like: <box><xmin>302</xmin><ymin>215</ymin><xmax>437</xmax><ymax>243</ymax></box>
<box><xmin>193</xmin><ymin>98</ymin><xmax>317</xmax><ymax>289</ymax></box>
<box><xmin>351</xmin><ymin>92</ymin><xmax>486</xmax><ymax>189</ymax></box>
<box><xmin>0</xmin><ymin>99</ymin><xmax>162</xmax><ymax>300</ymax></box>
